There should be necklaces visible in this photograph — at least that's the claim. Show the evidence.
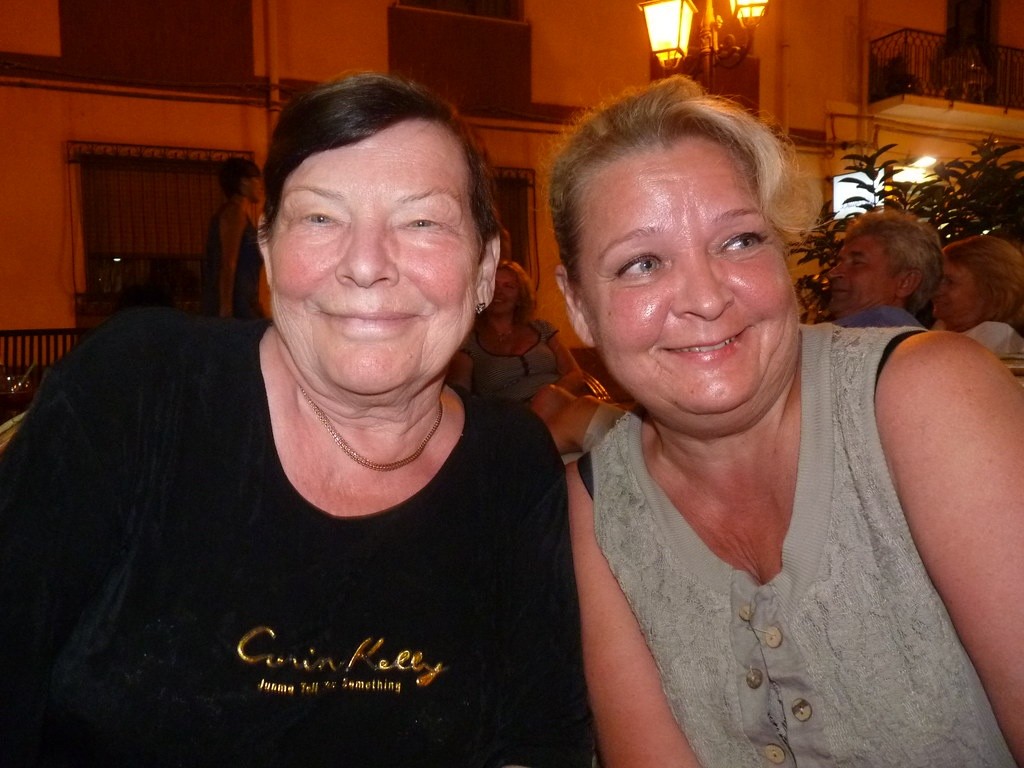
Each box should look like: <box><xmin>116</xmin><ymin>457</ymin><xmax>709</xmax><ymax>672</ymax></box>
<box><xmin>301</xmin><ymin>388</ymin><xmax>443</xmax><ymax>472</ymax></box>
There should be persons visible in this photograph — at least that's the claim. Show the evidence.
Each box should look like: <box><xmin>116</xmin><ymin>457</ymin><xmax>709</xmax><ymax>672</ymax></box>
<box><xmin>930</xmin><ymin>234</ymin><xmax>1023</xmax><ymax>359</ymax></box>
<box><xmin>550</xmin><ymin>76</ymin><xmax>1023</xmax><ymax>767</ymax></box>
<box><xmin>818</xmin><ymin>209</ymin><xmax>944</xmax><ymax>330</ymax></box>
<box><xmin>447</xmin><ymin>259</ymin><xmax>584</xmax><ymax>406</ymax></box>
<box><xmin>202</xmin><ymin>154</ymin><xmax>266</xmax><ymax>316</ymax></box>
<box><xmin>529</xmin><ymin>384</ymin><xmax>628</xmax><ymax>453</ymax></box>
<box><xmin>1</xmin><ymin>72</ymin><xmax>596</xmax><ymax>766</ymax></box>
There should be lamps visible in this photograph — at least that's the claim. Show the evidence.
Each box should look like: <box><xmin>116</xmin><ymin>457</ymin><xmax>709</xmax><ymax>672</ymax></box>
<box><xmin>641</xmin><ymin>0</ymin><xmax>770</xmax><ymax>98</ymax></box>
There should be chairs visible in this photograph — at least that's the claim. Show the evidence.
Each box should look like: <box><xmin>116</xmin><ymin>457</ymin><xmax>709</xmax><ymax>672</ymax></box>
<box><xmin>582</xmin><ymin>367</ymin><xmax>617</xmax><ymax>409</ymax></box>
